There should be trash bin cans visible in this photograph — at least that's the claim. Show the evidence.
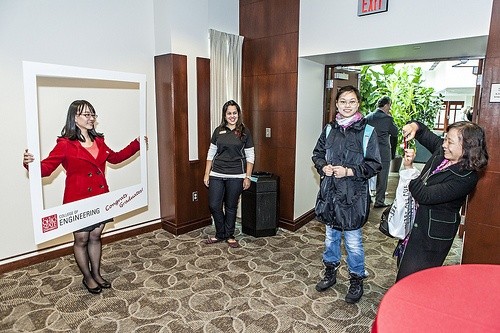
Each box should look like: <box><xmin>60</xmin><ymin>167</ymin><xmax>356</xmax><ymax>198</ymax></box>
<box><xmin>242</xmin><ymin>172</ymin><xmax>279</xmax><ymax>238</ymax></box>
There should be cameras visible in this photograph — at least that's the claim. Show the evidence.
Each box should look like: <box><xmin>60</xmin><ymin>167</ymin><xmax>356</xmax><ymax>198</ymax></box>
<box><xmin>400</xmin><ymin>137</ymin><xmax>408</xmax><ymax>150</ymax></box>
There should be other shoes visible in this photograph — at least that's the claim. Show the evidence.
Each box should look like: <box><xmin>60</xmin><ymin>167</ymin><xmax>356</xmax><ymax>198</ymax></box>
<box><xmin>204</xmin><ymin>237</ymin><xmax>223</xmax><ymax>244</ymax></box>
<box><xmin>374</xmin><ymin>204</ymin><xmax>387</xmax><ymax>207</ymax></box>
<box><xmin>227</xmin><ymin>239</ymin><xmax>239</xmax><ymax>248</ymax></box>
<box><xmin>82</xmin><ymin>276</ymin><xmax>111</xmax><ymax>293</ymax></box>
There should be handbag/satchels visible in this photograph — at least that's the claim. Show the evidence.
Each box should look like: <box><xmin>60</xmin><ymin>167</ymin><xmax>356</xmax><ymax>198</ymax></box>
<box><xmin>387</xmin><ymin>158</ymin><xmax>421</xmax><ymax>240</ymax></box>
<box><xmin>379</xmin><ymin>206</ymin><xmax>395</xmax><ymax>238</ymax></box>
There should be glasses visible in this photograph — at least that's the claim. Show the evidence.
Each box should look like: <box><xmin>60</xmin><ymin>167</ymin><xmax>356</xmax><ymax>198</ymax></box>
<box><xmin>76</xmin><ymin>114</ymin><xmax>98</xmax><ymax>119</ymax></box>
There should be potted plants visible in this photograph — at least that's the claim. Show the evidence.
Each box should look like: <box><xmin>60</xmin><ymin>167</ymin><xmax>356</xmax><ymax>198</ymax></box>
<box><xmin>354</xmin><ymin>62</ymin><xmax>446</xmax><ymax>172</ymax></box>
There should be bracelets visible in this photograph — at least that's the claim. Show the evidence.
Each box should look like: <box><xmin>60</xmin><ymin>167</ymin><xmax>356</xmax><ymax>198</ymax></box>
<box><xmin>345</xmin><ymin>167</ymin><xmax>347</xmax><ymax>177</ymax></box>
<box><xmin>245</xmin><ymin>176</ymin><xmax>251</xmax><ymax>181</ymax></box>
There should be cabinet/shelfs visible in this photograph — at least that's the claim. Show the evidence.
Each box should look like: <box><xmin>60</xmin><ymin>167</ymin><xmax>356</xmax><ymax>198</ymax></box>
<box><xmin>155</xmin><ymin>54</ymin><xmax>212</xmax><ymax>236</ymax></box>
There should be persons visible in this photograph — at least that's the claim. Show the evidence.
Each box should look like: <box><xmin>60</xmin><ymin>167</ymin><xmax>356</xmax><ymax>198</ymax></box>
<box><xmin>23</xmin><ymin>100</ymin><xmax>148</xmax><ymax>294</ymax></box>
<box><xmin>467</xmin><ymin>107</ymin><xmax>479</xmax><ymax>124</ymax></box>
<box><xmin>396</xmin><ymin>120</ymin><xmax>490</xmax><ymax>284</ymax></box>
<box><xmin>204</xmin><ymin>100</ymin><xmax>255</xmax><ymax>248</ymax></box>
<box><xmin>312</xmin><ymin>85</ymin><xmax>382</xmax><ymax>304</ymax></box>
<box><xmin>364</xmin><ymin>97</ymin><xmax>397</xmax><ymax>208</ymax></box>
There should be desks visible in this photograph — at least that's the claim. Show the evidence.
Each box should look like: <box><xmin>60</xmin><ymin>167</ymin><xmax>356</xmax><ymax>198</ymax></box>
<box><xmin>371</xmin><ymin>264</ymin><xmax>500</xmax><ymax>333</ymax></box>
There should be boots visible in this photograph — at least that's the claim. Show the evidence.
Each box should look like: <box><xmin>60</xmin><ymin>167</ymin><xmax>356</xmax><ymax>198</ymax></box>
<box><xmin>315</xmin><ymin>262</ymin><xmax>341</xmax><ymax>291</ymax></box>
<box><xmin>345</xmin><ymin>268</ymin><xmax>367</xmax><ymax>303</ymax></box>
